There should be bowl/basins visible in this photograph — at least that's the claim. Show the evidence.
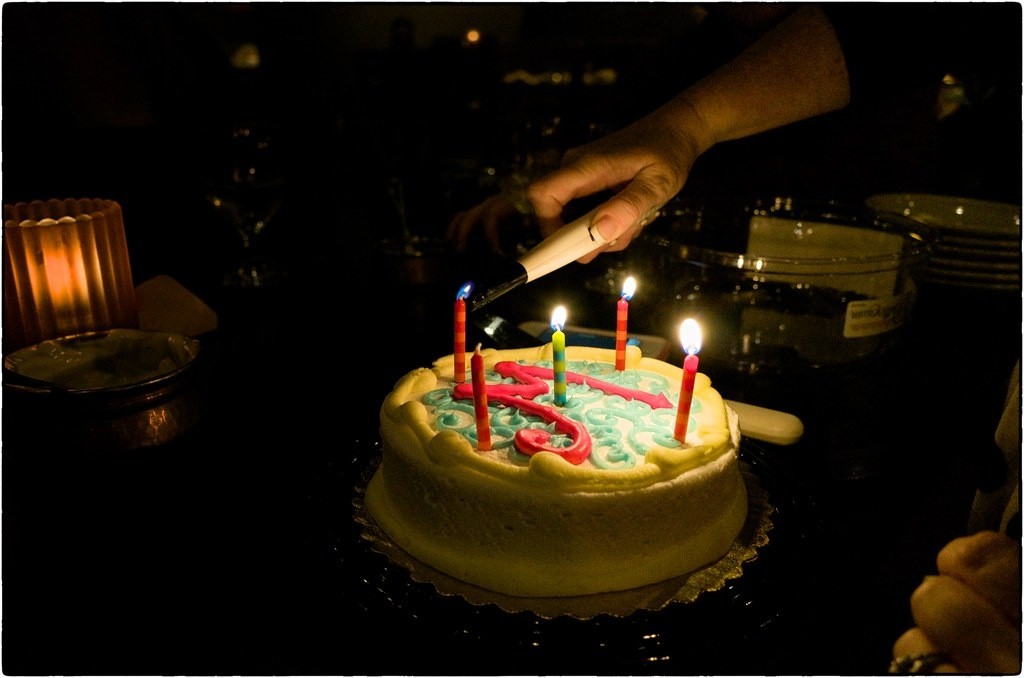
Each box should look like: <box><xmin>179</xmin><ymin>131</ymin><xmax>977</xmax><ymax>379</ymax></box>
<box><xmin>629</xmin><ymin>196</ymin><xmax>940</xmax><ymax>371</ymax></box>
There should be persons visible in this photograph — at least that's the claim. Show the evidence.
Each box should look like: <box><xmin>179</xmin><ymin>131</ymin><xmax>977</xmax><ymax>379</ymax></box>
<box><xmin>446</xmin><ymin>2</ymin><xmax>1022</xmax><ymax>264</ymax></box>
<box><xmin>886</xmin><ymin>532</ymin><xmax>1022</xmax><ymax>676</ymax></box>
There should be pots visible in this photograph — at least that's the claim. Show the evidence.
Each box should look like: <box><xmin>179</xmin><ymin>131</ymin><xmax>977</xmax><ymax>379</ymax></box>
<box><xmin>2</xmin><ymin>329</ymin><xmax>202</xmax><ymax>412</ymax></box>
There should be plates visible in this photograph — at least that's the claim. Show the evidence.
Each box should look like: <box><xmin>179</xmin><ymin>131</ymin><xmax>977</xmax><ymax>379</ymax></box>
<box><xmin>865</xmin><ymin>193</ymin><xmax>1020</xmax><ymax>291</ymax></box>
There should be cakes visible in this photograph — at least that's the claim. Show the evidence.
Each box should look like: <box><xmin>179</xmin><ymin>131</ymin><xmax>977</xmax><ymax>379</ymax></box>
<box><xmin>364</xmin><ymin>344</ymin><xmax>747</xmax><ymax>598</ymax></box>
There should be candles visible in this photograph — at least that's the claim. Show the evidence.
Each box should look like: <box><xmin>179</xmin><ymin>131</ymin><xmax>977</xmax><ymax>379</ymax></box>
<box><xmin>546</xmin><ymin>300</ymin><xmax>570</xmax><ymax>404</ymax></box>
<box><xmin>615</xmin><ymin>274</ymin><xmax>639</xmax><ymax>371</ymax></box>
<box><xmin>470</xmin><ymin>342</ymin><xmax>493</xmax><ymax>452</ymax></box>
<box><xmin>454</xmin><ymin>282</ymin><xmax>472</xmax><ymax>382</ymax></box>
<box><xmin>675</xmin><ymin>316</ymin><xmax>707</xmax><ymax>440</ymax></box>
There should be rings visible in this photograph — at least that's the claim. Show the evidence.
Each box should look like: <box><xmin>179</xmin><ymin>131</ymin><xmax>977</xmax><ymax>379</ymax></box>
<box><xmin>888</xmin><ymin>651</ymin><xmax>950</xmax><ymax>673</ymax></box>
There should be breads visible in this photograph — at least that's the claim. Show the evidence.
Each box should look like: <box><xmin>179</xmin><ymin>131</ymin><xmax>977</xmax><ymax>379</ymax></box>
<box><xmin>888</xmin><ymin>530</ymin><xmax>1022</xmax><ymax>675</ymax></box>
